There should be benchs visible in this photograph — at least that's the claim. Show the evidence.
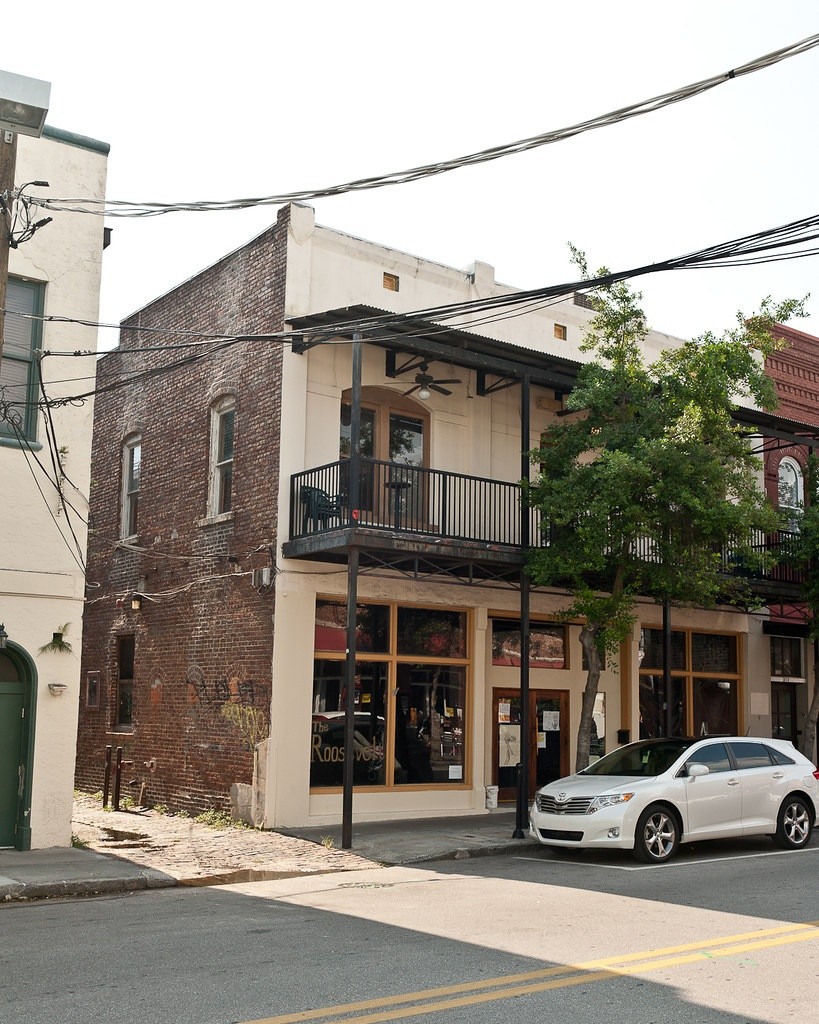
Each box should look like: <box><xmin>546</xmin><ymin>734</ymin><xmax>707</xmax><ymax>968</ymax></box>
<box><xmin>439</xmin><ymin>732</ymin><xmax>462</xmax><ymax>756</ymax></box>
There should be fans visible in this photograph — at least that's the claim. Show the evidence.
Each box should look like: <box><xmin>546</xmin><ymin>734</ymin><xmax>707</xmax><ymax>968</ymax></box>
<box><xmin>384</xmin><ymin>354</ymin><xmax>462</xmax><ymax>396</ymax></box>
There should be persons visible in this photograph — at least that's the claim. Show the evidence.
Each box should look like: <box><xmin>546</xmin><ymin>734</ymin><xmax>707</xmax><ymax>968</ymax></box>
<box><xmin>395</xmin><ymin>687</ymin><xmax>414</xmax><ymax>768</ymax></box>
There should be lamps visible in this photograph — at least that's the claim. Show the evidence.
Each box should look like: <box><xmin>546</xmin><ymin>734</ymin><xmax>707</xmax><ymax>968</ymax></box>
<box><xmin>47</xmin><ymin>683</ymin><xmax>67</xmax><ymax>696</ymax></box>
<box><xmin>132</xmin><ymin>593</ymin><xmax>143</xmax><ymax>610</ymax></box>
<box><xmin>418</xmin><ymin>383</ymin><xmax>431</xmax><ymax>400</ymax></box>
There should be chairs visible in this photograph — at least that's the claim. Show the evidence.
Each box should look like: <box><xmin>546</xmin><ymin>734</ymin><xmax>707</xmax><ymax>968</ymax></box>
<box><xmin>300</xmin><ymin>485</ymin><xmax>342</xmax><ymax>535</ymax></box>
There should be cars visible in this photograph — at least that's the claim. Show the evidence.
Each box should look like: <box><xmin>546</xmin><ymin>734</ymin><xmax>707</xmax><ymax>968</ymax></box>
<box><xmin>527</xmin><ymin>736</ymin><xmax>819</xmax><ymax>864</ymax></box>
<box><xmin>312</xmin><ymin>711</ymin><xmax>384</xmax><ymax>788</ymax></box>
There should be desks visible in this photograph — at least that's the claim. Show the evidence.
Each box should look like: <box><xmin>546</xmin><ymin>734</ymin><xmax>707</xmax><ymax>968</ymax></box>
<box><xmin>382</xmin><ymin>482</ymin><xmax>412</xmax><ymax>529</ymax></box>
